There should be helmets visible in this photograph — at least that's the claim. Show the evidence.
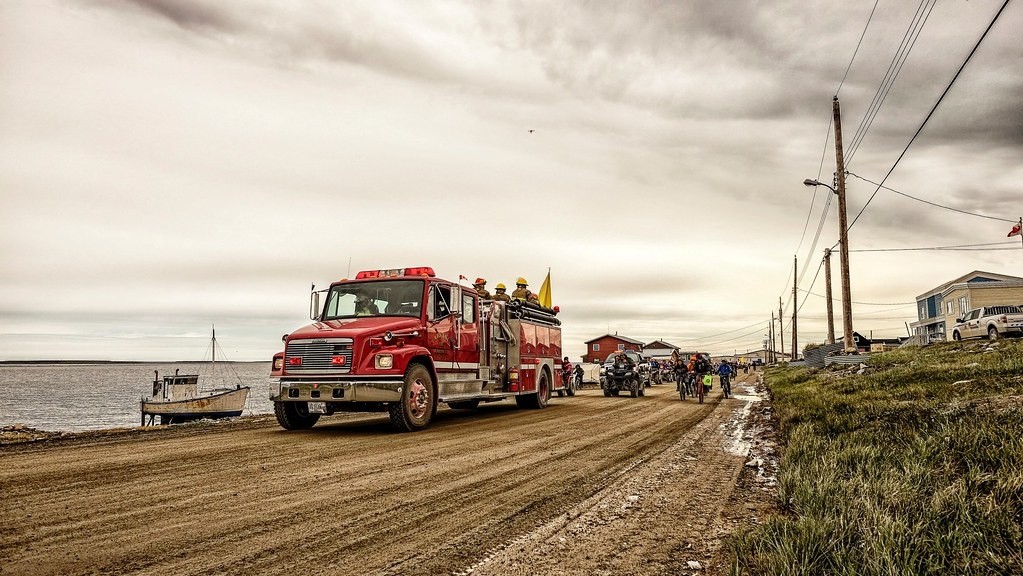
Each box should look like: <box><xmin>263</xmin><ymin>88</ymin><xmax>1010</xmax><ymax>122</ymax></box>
<box><xmin>475</xmin><ymin>279</ymin><xmax>485</xmax><ymax>285</ymax></box>
<box><xmin>516</xmin><ymin>278</ymin><xmax>529</xmax><ymax>286</ymax></box>
<box><xmin>359</xmin><ymin>296</ymin><xmax>371</xmax><ymax>311</ymax></box>
<box><xmin>495</xmin><ymin>284</ymin><xmax>506</xmax><ymax>290</ymax></box>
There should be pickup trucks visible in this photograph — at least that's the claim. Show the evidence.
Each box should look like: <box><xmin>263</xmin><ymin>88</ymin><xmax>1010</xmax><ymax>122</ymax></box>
<box><xmin>952</xmin><ymin>305</ymin><xmax>1023</xmax><ymax>342</ymax></box>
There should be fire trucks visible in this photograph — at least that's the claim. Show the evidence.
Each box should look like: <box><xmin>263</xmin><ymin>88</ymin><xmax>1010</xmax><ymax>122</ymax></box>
<box><xmin>269</xmin><ymin>265</ymin><xmax>568</xmax><ymax>433</ymax></box>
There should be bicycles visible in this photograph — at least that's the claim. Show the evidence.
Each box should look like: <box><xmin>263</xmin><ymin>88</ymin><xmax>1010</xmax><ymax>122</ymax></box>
<box><xmin>573</xmin><ymin>349</ymin><xmax>757</xmax><ymax>404</ymax></box>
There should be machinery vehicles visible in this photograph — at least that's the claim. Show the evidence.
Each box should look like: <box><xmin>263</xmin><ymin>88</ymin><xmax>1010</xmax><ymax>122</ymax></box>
<box><xmin>604</xmin><ymin>352</ymin><xmax>646</xmax><ymax>397</ymax></box>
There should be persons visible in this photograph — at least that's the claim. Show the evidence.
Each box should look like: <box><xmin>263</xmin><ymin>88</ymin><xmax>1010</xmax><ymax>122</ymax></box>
<box><xmin>662</xmin><ymin>360</ymin><xmax>673</xmax><ymax>370</ymax></box>
<box><xmin>613</xmin><ymin>353</ymin><xmax>636</xmax><ymax>369</ymax></box>
<box><xmin>573</xmin><ymin>364</ymin><xmax>585</xmax><ymax>388</ymax></box>
<box><xmin>354</xmin><ymin>294</ymin><xmax>380</xmax><ymax>314</ymax></box>
<box><xmin>715</xmin><ymin>360</ymin><xmax>733</xmax><ymax>395</ymax></box>
<box><xmin>562</xmin><ymin>357</ymin><xmax>572</xmax><ymax>386</ymax></box>
<box><xmin>473</xmin><ymin>278</ymin><xmax>490</xmax><ymax>300</ymax></box>
<box><xmin>512</xmin><ymin>278</ymin><xmax>531</xmax><ymax>302</ymax></box>
<box><xmin>674</xmin><ymin>353</ymin><xmax>712</xmax><ymax>396</ymax></box>
<box><xmin>492</xmin><ymin>284</ymin><xmax>511</xmax><ymax>301</ymax></box>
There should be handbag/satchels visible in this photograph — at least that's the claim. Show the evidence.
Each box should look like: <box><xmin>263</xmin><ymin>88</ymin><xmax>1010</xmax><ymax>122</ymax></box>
<box><xmin>703</xmin><ymin>374</ymin><xmax>712</xmax><ymax>386</ymax></box>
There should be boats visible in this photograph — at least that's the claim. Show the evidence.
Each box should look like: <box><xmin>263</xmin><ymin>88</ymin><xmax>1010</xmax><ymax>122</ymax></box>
<box><xmin>138</xmin><ymin>323</ymin><xmax>252</xmax><ymax>417</ymax></box>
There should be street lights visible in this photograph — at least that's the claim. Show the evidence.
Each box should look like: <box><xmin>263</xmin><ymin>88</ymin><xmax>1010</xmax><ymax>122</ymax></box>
<box><xmin>803</xmin><ymin>176</ymin><xmax>854</xmax><ymax>353</ymax></box>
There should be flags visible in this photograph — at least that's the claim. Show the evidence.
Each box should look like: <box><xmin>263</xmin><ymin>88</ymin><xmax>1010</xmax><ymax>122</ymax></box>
<box><xmin>1007</xmin><ymin>220</ymin><xmax>1022</xmax><ymax>237</ymax></box>
